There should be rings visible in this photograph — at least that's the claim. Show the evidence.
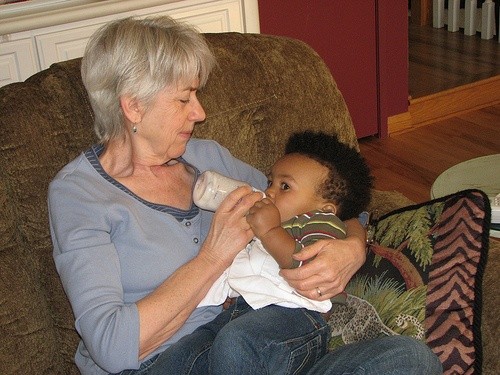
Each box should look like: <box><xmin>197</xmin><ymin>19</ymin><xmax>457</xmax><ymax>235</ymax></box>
<box><xmin>316</xmin><ymin>287</ymin><xmax>321</xmax><ymax>296</ymax></box>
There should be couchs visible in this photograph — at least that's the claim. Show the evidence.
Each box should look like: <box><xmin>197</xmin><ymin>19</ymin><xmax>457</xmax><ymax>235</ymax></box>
<box><xmin>0</xmin><ymin>33</ymin><xmax>500</xmax><ymax>375</ymax></box>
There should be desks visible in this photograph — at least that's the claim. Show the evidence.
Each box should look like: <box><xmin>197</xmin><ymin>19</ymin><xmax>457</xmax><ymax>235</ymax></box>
<box><xmin>429</xmin><ymin>154</ymin><xmax>500</xmax><ymax>237</ymax></box>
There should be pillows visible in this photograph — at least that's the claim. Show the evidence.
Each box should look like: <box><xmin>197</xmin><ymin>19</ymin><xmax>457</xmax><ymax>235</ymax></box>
<box><xmin>329</xmin><ymin>189</ymin><xmax>492</xmax><ymax>375</ymax></box>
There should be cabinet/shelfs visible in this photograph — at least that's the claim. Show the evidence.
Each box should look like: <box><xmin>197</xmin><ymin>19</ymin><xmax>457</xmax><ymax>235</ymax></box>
<box><xmin>0</xmin><ymin>0</ymin><xmax>259</xmax><ymax>90</ymax></box>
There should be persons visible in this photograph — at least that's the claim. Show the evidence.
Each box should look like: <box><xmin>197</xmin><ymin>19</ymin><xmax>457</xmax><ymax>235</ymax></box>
<box><xmin>48</xmin><ymin>14</ymin><xmax>441</xmax><ymax>375</ymax></box>
<box><xmin>140</xmin><ymin>131</ymin><xmax>371</xmax><ymax>375</ymax></box>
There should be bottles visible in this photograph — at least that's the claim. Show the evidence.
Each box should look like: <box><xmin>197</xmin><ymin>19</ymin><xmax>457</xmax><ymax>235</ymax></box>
<box><xmin>193</xmin><ymin>170</ymin><xmax>267</xmax><ymax>212</ymax></box>
